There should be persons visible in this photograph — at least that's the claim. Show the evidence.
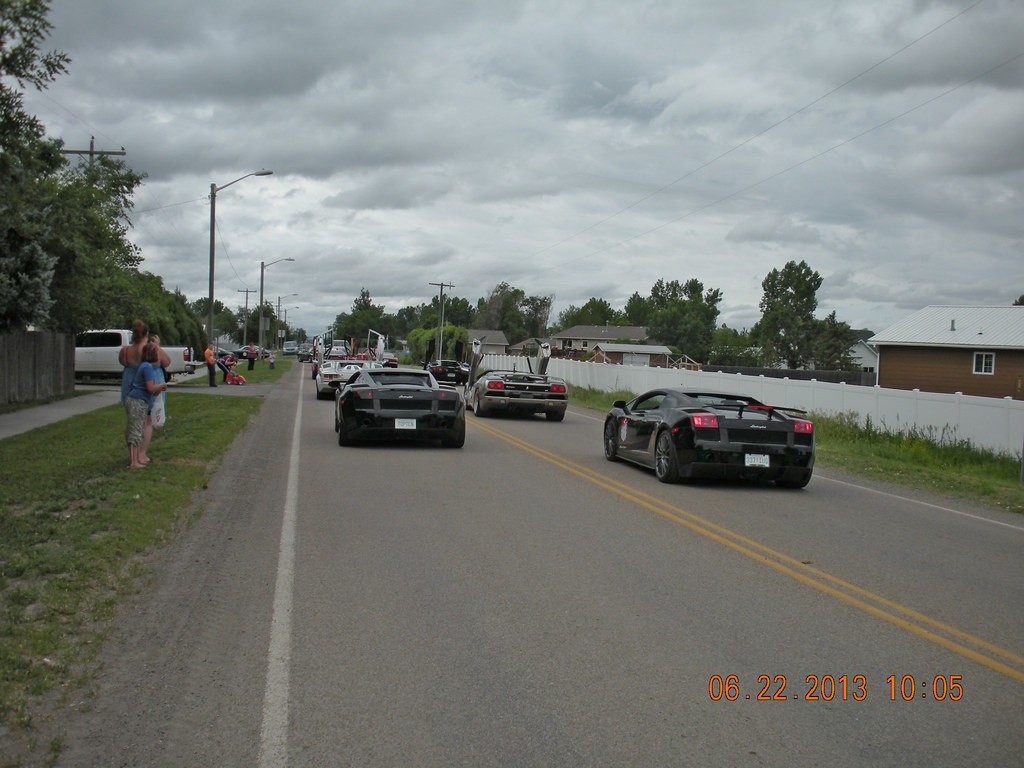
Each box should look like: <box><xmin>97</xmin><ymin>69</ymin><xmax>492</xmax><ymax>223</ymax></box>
<box><xmin>119</xmin><ymin>318</ymin><xmax>171</xmax><ymax>462</ymax></box>
<box><xmin>270</xmin><ymin>352</ymin><xmax>276</xmax><ymax>369</ymax></box>
<box><xmin>229</xmin><ymin>365</ymin><xmax>237</xmax><ymax>378</ymax></box>
<box><xmin>247</xmin><ymin>341</ymin><xmax>256</xmax><ymax>371</ymax></box>
<box><xmin>217</xmin><ymin>355</ymin><xmax>238</xmax><ymax>382</ymax></box>
<box><xmin>125</xmin><ymin>342</ymin><xmax>168</xmax><ymax>469</ymax></box>
<box><xmin>205</xmin><ymin>342</ymin><xmax>218</xmax><ymax>387</ymax></box>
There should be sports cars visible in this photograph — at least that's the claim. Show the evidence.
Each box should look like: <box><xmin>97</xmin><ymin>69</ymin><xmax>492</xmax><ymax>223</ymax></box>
<box><xmin>604</xmin><ymin>388</ymin><xmax>815</xmax><ymax>489</ymax></box>
<box><xmin>420</xmin><ymin>338</ymin><xmax>470</xmax><ymax>386</ymax></box>
<box><xmin>313</xmin><ymin>328</ymin><xmax>398</xmax><ymax>400</ymax></box>
<box><xmin>464</xmin><ymin>335</ymin><xmax>568</xmax><ymax>422</ymax></box>
<box><xmin>328</xmin><ymin>369</ymin><xmax>466</xmax><ymax>449</ymax></box>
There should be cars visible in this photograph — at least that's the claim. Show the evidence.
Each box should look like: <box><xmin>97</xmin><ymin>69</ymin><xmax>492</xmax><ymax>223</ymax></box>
<box><xmin>233</xmin><ymin>345</ymin><xmax>273</xmax><ymax>359</ymax></box>
<box><xmin>296</xmin><ymin>339</ymin><xmax>400</xmax><ymax>368</ymax></box>
<box><xmin>212</xmin><ymin>347</ymin><xmax>235</xmax><ymax>363</ymax></box>
<box><xmin>461</xmin><ymin>362</ymin><xmax>472</xmax><ymax>372</ymax></box>
<box><xmin>226</xmin><ymin>373</ymin><xmax>247</xmax><ymax>385</ymax></box>
<box><xmin>283</xmin><ymin>341</ymin><xmax>298</xmax><ymax>355</ymax></box>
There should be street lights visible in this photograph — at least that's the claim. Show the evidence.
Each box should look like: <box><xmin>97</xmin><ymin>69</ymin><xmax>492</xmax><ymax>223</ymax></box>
<box><xmin>258</xmin><ymin>256</ymin><xmax>295</xmax><ymax>361</ymax></box>
<box><xmin>207</xmin><ymin>168</ymin><xmax>275</xmax><ymax>366</ymax></box>
<box><xmin>277</xmin><ymin>293</ymin><xmax>300</xmax><ymax>354</ymax></box>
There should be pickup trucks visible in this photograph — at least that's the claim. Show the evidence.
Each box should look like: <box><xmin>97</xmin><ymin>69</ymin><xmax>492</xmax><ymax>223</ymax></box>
<box><xmin>73</xmin><ymin>329</ymin><xmax>196</xmax><ymax>385</ymax></box>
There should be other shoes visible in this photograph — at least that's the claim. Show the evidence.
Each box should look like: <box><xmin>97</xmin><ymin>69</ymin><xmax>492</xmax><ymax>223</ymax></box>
<box><xmin>126</xmin><ymin>463</ymin><xmax>146</xmax><ymax>469</ymax></box>
<box><xmin>138</xmin><ymin>457</ymin><xmax>150</xmax><ymax>463</ymax></box>
